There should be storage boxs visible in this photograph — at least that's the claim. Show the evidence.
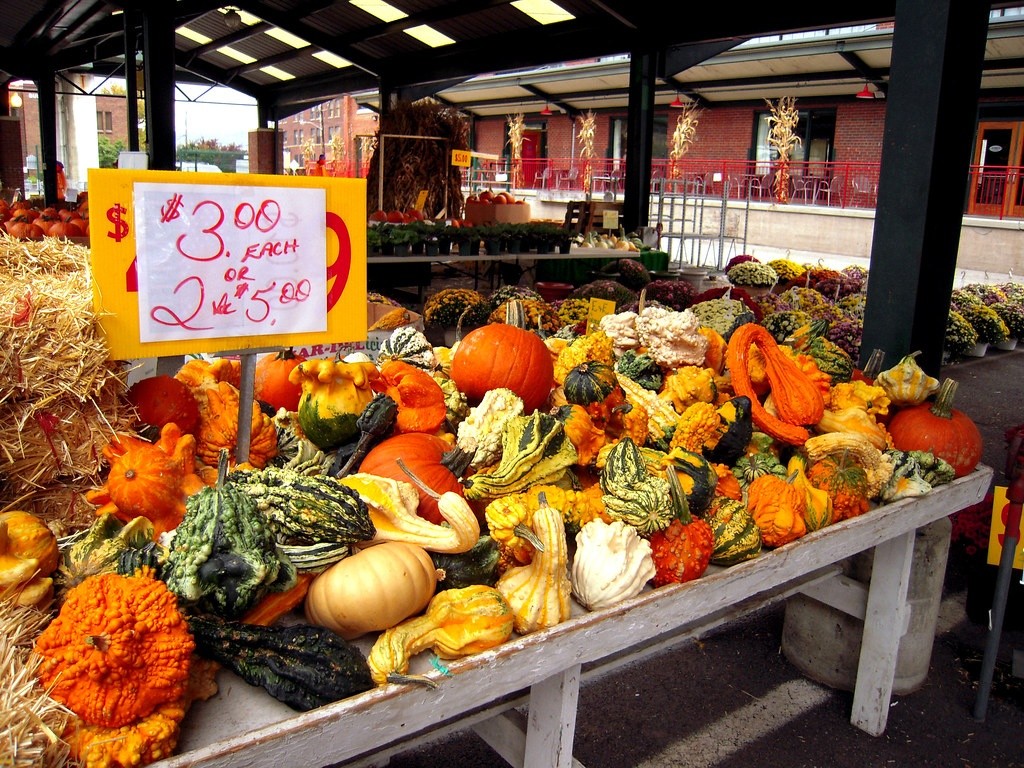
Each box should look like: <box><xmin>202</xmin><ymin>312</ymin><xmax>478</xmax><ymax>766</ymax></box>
<box><xmin>284</xmin><ymin>303</ymin><xmax>425</xmax><ymax>363</ymax></box>
<box><xmin>466</xmin><ymin>202</ymin><xmax>531</xmax><ymax>227</ymax></box>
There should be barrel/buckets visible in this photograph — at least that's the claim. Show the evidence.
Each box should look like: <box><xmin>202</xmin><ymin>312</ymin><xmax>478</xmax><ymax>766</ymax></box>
<box><xmin>781</xmin><ymin>516</ymin><xmax>952</xmax><ymax>696</ymax></box>
<box><xmin>678</xmin><ymin>269</ymin><xmax>707</xmax><ymax>290</ymax></box>
<box><xmin>118</xmin><ymin>151</ymin><xmax>148</xmax><ymax>170</ymax></box>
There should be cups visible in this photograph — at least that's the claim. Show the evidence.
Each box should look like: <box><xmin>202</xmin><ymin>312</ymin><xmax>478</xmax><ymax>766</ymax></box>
<box><xmin>850</xmin><ymin>368</ymin><xmax>873</xmax><ymax>386</ymax></box>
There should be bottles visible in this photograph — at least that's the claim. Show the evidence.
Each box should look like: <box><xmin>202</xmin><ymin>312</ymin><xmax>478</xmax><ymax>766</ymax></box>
<box><xmin>861</xmin><ymin>349</ymin><xmax>885</xmax><ymax>381</ymax></box>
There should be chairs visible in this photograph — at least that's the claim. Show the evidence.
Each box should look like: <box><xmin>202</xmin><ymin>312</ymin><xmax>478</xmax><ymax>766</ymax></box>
<box><xmin>533</xmin><ymin>176</ymin><xmax>879</xmax><ymax>210</ymax></box>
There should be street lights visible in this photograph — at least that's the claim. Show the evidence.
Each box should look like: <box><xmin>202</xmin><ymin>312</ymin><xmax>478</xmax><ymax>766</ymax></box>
<box><xmin>300</xmin><ymin>121</ymin><xmax>325</xmax><ymax>162</ymax></box>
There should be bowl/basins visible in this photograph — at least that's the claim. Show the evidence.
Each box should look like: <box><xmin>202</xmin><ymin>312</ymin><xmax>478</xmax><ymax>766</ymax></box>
<box><xmin>648</xmin><ymin>270</ymin><xmax>680</xmax><ymax>282</ymax></box>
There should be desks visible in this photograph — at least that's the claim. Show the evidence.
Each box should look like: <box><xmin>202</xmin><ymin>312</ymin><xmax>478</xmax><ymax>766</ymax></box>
<box><xmin>635</xmin><ymin>251</ymin><xmax>669</xmax><ymax>273</ymax></box>
<box><xmin>549</xmin><ymin>169</ymin><xmax>570</xmax><ymax>189</ymax></box>
<box><xmin>135</xmin><ymin>463</ymin><xmax>995</xmax><ymax>768</ymax></box>
<box><xmin>686</xmin><ymin>171</ymin><xmax>706</xmax><ymax>193</ymax></box>
<box><xmin>800</xmin><ymin>174</ymin><xmax>830</xmax><ymax>205</ymax></box>
<box><xmin>367</xmin><ymin>253</ymin><xmax>641</xmax><ymax>306</ymax></box>
<box><xmin>740</xmin><ymin>173</ymin><xmax>758</xmax><ymax>200</ymax></box>
<box><xmin>595</xmin><ymin>170</ymin><xmax>611</xmax><ymax>191</ymax></box>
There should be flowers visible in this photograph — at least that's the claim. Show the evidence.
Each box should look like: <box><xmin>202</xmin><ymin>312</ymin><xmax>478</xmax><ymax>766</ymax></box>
<box><xmin>727</xmin><ymin>262</ymin><xmax>779</xmax><ymax>287</ymax></box>
<box><xmin>419</xmin><ymin>285</ymin><xmax>492</xmax><ymax>330</ymax></box>
<box><xmin>944</xmin><ymin>282</ymin><xmax>1024</xmax><ymax>353</ymax></box>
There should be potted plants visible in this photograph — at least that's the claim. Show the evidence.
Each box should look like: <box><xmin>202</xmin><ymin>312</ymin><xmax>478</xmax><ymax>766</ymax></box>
<box><xmin>365</xmin><ymin>222</ymin><xmax>578</xmax><ymax>254</ymax></box>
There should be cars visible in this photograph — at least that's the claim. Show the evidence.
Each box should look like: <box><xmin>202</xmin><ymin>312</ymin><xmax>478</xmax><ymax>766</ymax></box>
<box><xmin>175</xmin><ymin>161</ymin><xmax>222</xmax><ymax>174</ymax></box>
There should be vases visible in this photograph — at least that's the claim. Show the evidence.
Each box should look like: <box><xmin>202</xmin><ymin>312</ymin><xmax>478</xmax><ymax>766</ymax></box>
<box><xmin>535</xmin><ymin>281</ymin><xmax>574</xmax><ymax>302</ymax></box>
<box><xmin>444</xmin><ymin>326</ymin><xmax>469</xmax><ymax>349</ymax></box>
<box><xmin>680</xmin><ymin>271</ymin><xmax>706</xmax><ymax>290</ymax></box>
<box><xmin>735</xmin><ymin>286</ymin><xmax>768</xmax><ymax>298</ymax></box>
<box><xmin>964</xmin><ymin>343</ymin><xmax>989</xmax><ymax>357</ymax></box>
<box><xmin>995</xmin><ymin>336</ymin><xmax>1018</xmax><ymax>350</ymax></box>
<box><xmin>587</xmin><ymin>270</ymin><xmax>622</xmax><ymax>284</ymax></box>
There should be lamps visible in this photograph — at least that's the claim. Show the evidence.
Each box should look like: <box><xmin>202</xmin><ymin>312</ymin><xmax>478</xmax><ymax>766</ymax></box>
<box><xmin>855</xmin><ymin>83</ymin><xmax>874</xmax><ymax>99</ymax></box>
<box><xmin>540</xmin><ymin>106</ymin><xmax>552</xmax><ymax>116</ymax></box>
<box><xmin>669</xmin><ymin>95</ymin><xmax>685</xmax><ymax>107</ymax></box>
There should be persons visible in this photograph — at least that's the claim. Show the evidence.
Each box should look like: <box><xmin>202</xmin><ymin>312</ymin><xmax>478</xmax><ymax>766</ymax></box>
<box><xmin>316</xmin><ymin>154</ymin><xmax>326</xmax><ymax>167</ymax></box>
<box><xmin>56</xmin><ymin>160</ymin><xmax>67</xmax><ymax>200</ymax></box>
